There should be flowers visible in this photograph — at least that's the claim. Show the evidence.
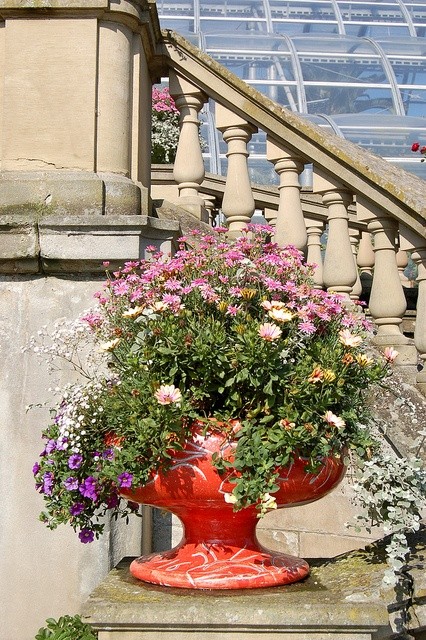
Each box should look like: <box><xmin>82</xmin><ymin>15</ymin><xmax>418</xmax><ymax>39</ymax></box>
<box><xmin>17</xmin><ymin>220</ymin><xmax>401</xmax><ymax>547</ymax></box>
<box><xmin>409</xmin><ymin>141</ymin><xmax>426</xmax><ymax>167</ymax></box>
<box><xmin>150</xmin><ymin>83</ymin><xmax>210</xmax><ymax>163</ymax></box>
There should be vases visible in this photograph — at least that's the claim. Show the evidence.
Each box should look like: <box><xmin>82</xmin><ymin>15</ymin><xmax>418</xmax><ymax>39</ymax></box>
<box><xmin>103</xmin><ymin>417</ymin><xmax>349</xmax><ymax>591</ymax></box>
